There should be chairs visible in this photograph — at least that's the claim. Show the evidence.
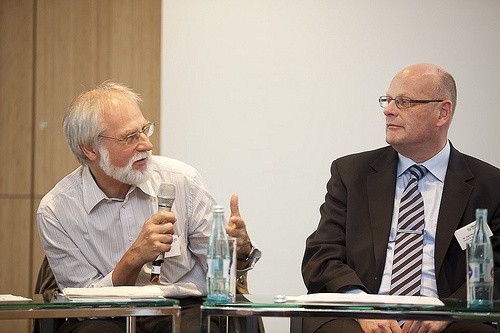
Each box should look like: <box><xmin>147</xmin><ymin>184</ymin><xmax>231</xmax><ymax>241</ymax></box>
<box><xmin>34</xmin><ymin>255</ymin><xmax>265</xmax><ymax>333</ymax></box>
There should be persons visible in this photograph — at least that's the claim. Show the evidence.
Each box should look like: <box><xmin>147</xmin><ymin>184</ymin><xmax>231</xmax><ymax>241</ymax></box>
<box><xmin>301</xmin><ymin>62</ymin><xmax>500</xmax><ymax>333</ymax></box>
<box><xmin>37</xmin><ymin>80</ymin><xmax>263</xmax><ymax>333</ymax></box>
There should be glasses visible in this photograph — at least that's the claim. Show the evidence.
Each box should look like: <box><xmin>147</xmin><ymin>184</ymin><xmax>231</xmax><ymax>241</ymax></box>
<box><xmin>379</xmin><ymin>96</ymin><xmax>444</xmax><ymax>110</ymax></box>
<box><xmin>98</xmin><ymin>121</ymin><xmax>155</xmax><ymax>147</ymax></box>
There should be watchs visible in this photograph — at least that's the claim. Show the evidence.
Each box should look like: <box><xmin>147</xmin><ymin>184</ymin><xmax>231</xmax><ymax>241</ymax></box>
<box><xmin>237</xmin><ymin>245</ymin><xmax>255</xmax><ymax>262</ymax></box>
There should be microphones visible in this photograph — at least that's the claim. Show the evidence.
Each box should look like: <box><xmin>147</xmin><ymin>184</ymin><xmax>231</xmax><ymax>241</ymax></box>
<box><xmin>150</xmin><ymin>183</ymin><xmax>175</xmax><ymax>285</ymax></box>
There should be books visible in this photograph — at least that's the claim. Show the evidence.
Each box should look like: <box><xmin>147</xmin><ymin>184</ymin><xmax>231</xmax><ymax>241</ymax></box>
<box><xmin>0</xmin><ymin>294</ymin><xmax>34</xmax><ymax>305</ymax></box>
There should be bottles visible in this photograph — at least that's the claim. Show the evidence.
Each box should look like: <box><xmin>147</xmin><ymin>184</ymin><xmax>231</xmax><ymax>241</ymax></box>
<box><xmin>205</xmin><ymin>206</ymin><xmax>230</xmax><ymax>303</ymax></box>
<box><xmin>468</xmin><ymin>208</ymin><xmax>493</xmax><ymax>309</ymax></box>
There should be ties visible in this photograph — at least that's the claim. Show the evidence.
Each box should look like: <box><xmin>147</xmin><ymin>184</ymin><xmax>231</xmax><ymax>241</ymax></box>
<box><xmin>390</xmin><ymin>164</ymin><xmax>429</xmax><ymax>328</ymax></box>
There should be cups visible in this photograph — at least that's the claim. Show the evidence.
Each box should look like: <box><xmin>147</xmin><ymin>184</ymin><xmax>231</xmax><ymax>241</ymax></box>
<box><xmin>465</xmin><ymin>242</ymin><xmax>486</xmax><ymax>308</ymax></box>
<box><xmin>215</xmin><ymin>237</ymin><xmax>238</xmax><ymax>302</ymax></box>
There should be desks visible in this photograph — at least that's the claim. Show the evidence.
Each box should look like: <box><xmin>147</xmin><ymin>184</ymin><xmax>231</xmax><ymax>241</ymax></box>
<box><xmin>0</xmin><ymin>294</ymin><xmax>206</xmax><ymax>333</ymax></box>
<box><xmin>199</xmin><ymin>290</ymin><xmax>499</xmax><ymax>333</ymax></box>
